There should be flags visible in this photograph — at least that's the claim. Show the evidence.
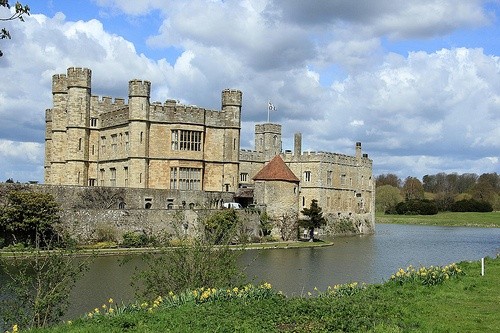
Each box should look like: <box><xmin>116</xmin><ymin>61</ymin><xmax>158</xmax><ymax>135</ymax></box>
<box><xmin>269</xmin><ymin>102</ymin><xmax>277</xmax><ymax>111</ymax></box>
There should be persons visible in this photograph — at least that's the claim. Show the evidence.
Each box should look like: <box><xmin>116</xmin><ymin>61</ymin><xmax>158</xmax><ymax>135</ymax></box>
<box><xmin>303</xmin><ymin>228</ymin><xmax>308</xmax><ymax>238</ymax></box>
